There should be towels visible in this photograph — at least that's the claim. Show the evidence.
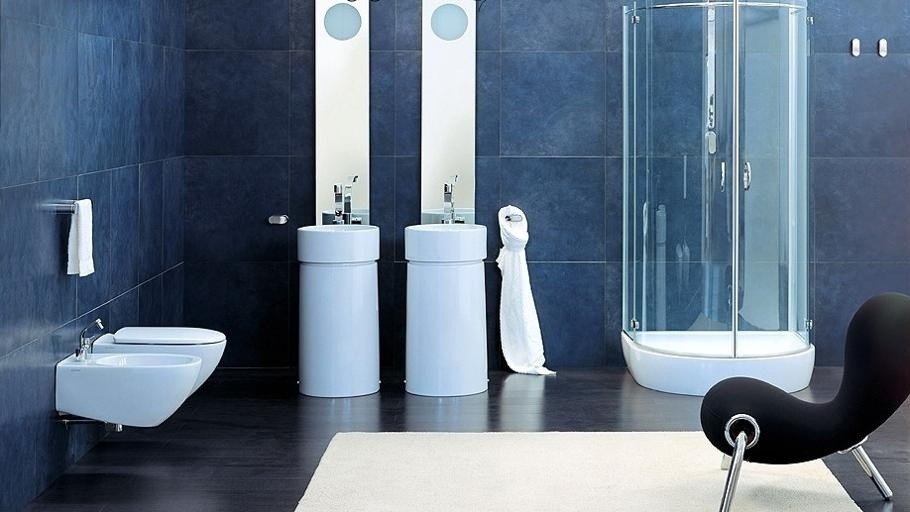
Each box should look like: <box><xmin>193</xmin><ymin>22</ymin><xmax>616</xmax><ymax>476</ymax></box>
<box><xmin>65</xmin><ymin>199</ymin><xmax>95</xmax><ymax>278</ymax></box>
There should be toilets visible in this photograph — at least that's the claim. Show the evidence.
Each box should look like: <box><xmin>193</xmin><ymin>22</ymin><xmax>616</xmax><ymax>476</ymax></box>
<box><xmin>91</xmin><ymin>326</ymin><xmax>228</xmax><ymax>400</ymax></box>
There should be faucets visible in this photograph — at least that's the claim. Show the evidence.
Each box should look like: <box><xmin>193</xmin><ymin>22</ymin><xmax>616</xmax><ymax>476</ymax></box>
<box><xmin>333</xmin><ymin>184</ymin><xmax>345</xmax><ymax>225</ymax></box>
<box><xmin>344</xmin><ymin>175</ymin><xmax>359</xmax><ymax>225</ymax></box>
<box><xmin>448</xmin><ymin>174</ymin><xmax>459</xmax><ymax>205</ymax></box>
<box><xmin>79</xmin><ymin>317</ymin><xmax>104</xmax><ymax>347</ymax></box>
<box><xmin>442</xmin><ymin>184</ymin><xmax>455</xmax><ymax>223</ymax></box>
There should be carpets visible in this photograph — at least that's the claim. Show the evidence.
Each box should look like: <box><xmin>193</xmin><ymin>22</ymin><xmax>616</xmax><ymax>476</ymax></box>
<box><xmin>293</xmin><ymin>430</ymin><xmax>864</xmax><ymax>510</ymax></box>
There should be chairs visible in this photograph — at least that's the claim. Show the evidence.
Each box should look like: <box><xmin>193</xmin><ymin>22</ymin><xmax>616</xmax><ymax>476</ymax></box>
<box><xmin>699</xmin><ymin>292</ymin><xmax>910</xmax><ymax>511</ymax></box>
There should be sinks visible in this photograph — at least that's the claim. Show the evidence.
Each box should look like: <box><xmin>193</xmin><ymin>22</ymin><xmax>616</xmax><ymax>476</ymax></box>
<box><xmin>405</xmin><ymin>223</ymin><xmax>488</xmax><ymax>261</ymax></box>
<box><xmin>55</xmin><ymin>352</ymin><xmax>203</xmax><ymax>428</ymax></box>
<box><xmin>295</xmin><ymin>224</ymin><xmax>380</xmax><ymax>263</ymax></box>
<box><xmin>322</xmin><ymin>209</ymin><xmax>369</xmax><ymax>225</ymax></box>
<box><xmin>421</xmin><ymin>207</ymin><xmax>474</xmax><ymax>224</ymax></box>
